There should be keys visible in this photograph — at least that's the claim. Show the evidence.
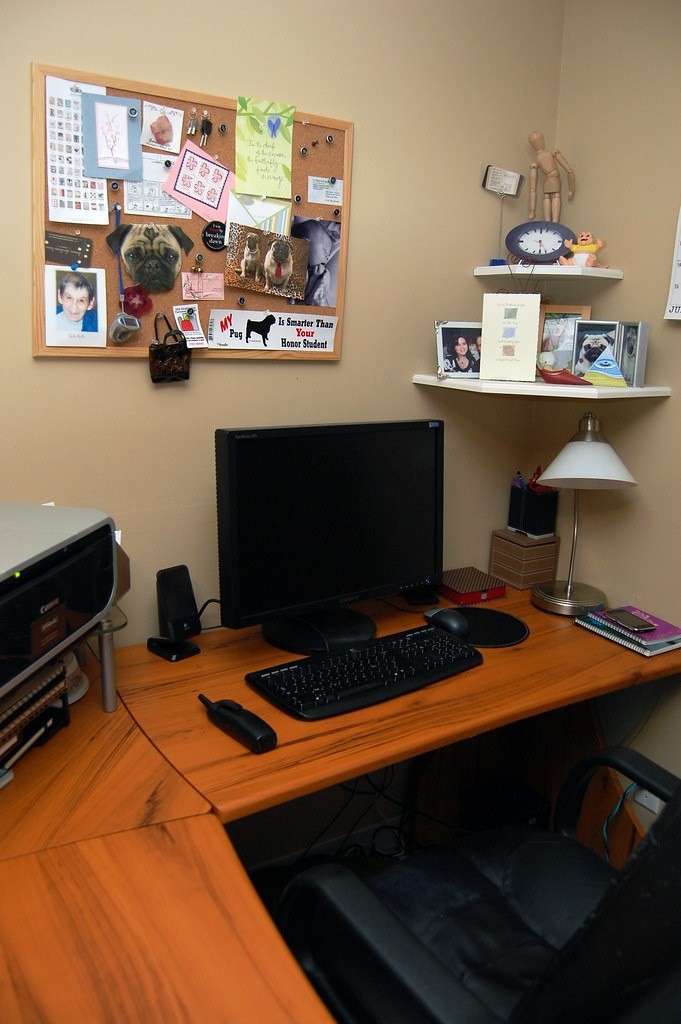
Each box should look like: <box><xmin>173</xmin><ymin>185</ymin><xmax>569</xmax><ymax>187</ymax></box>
<box><xmin>187</xmin><ymin>118</ymin><xmax>197</xmax><ymax>134</ymax></box>
<box><xmin>200</xmin><ymin>119</ymin><xmax>213</xmax><ymax>147</ymax></box>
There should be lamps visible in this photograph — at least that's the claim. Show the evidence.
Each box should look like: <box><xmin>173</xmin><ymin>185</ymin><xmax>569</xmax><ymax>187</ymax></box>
<box><xmin>530</xmin><ymin>412</ymin><xmax>639</xmax><ymax>615</ymax></box>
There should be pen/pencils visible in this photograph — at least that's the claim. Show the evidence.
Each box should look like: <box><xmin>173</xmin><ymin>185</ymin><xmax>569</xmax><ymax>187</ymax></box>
<box><xmin>512</xmin><ymin>470</ymin><xmax>526</xmax><ymax>490</ymax></box>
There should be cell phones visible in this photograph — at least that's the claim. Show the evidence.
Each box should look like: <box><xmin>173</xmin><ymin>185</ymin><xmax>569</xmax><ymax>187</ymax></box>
<box><xmin>603</xmin><ymin>609</ymin><xmax>656</xmax><ymax>633</ymax></box>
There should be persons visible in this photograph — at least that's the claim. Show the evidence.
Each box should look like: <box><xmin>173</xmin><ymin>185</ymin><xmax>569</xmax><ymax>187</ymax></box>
<box><xmin>443</xmin><ymin>335</ymin><xmax>482</xmax><ymax>373</ymax></box>
<box><xmin>559</xmin><ymin>232</ymin><xmax>606</xmax><ymax>267</ymax></box>
<box><xmin>528</xmin><ymin>131</ymin><xmax>575</xmax><ymax>223</ymax></box>
<box><xmin>56</xmin><ymin>271</ymin><xmax>97</xmax><ymax>332</ymax></box>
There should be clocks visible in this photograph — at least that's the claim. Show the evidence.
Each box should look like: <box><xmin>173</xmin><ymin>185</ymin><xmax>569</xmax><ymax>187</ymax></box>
<box><xmin>506</xmin><ymin>220</ymin><xmax>576</xmax><ymax>262</ymax></box>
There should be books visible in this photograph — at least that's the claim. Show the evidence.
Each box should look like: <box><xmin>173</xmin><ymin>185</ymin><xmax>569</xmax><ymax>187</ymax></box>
<box><xmin>588</xmin><ymin>605</ymin><xmax>681</xmax><ymax>645</ymax></box>
<box><xmin>574</xmin><ymin>615</ymin><xmax>681</xmax><ymax>656</ymax></box>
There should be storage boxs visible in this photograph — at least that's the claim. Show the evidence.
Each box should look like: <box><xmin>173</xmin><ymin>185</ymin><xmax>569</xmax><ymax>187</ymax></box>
<box><xmin>490</xmin><ymin>528</ymin><xmax>562</xmax><ymax>591</ymax></box>
<box><xmin>506</xmin><ymin>486</ymin><xmax>559</xmax><ymax>539</ymax></box>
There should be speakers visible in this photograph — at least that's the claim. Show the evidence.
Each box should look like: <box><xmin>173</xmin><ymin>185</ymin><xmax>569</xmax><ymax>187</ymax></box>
<box><xmin>156</xmin><ymin>563</ymin><xmax>202</xmax><ymax>641</ymax></box>
<box><xmin>461</xmin><ymin>776</ymin><xmax>553</xmax><ymax>834</ymax></box>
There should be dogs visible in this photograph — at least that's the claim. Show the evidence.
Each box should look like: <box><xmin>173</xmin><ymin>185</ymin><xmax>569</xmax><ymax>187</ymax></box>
<box><xmin>106</xmin><ymin>224</ymin><xmax>194</xmax><ymax>293</ymax></box>
<box><xmin>233</xmin><ymin>232</ymin><xmax>294</xmax><ymax>294</ymax></box>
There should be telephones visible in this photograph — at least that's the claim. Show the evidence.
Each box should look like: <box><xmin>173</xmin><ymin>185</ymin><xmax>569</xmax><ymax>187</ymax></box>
<box><xmin>197</xmin><ymin>692</ymin><xmax>277</xmax><ymax>755</ymax></box>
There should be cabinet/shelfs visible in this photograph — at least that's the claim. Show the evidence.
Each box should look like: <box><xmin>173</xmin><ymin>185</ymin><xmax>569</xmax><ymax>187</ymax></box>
<box><xmin>413</xmin><ymin>262</ymin><xmax>673</xmax><ymax>400</ymax></box>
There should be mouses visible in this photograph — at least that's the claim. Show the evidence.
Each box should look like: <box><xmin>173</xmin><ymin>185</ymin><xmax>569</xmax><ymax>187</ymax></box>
<box><xmin>424</xmin><ymin>609</ymin><xmax>468</xmax><ymax>637</ymax></box>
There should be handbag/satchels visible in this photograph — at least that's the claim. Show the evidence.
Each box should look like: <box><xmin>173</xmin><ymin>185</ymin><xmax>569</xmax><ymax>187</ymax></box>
<box><xmin>149</xmin><ymin>312</ymin><xmax>190</xmax><ymax>383</ymax></box>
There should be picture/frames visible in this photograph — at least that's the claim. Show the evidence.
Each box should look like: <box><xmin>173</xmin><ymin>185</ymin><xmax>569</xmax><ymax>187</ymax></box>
<box><xmin>618</xmin><ymin>320</ymin><xmax>650</xmax><ymax>386</ymax></box>
<box><xmin>537</xmin><ymin>304</ymin><xmax>592</xmax><ymax>376</ymax></box>
<box><xmin>434</xmin><ymin>319</ymin><xmax>482</xmax><ymax>380</ymax></box>
<box><xmin>43</xmin><ymin>264</ymin><xmax>108</xmax><ymax>349</ymax></box>
<box><xmin>573</xmin><ymin>319</ymin><xmax>620</xmax><ymax>379</ymax></box>
<box><xmin>30</xmin><ymin>61</ymin><xmax>356</xmax><ymax>361</ymax></box>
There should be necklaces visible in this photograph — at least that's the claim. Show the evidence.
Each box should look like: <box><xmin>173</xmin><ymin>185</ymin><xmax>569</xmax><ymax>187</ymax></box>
<box><xmin>458</xmin><ymin>359</ymin><xmax>468</xmax><ymax>364</ymax></box>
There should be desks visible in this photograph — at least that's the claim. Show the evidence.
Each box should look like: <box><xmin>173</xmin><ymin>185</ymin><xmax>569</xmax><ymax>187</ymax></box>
<box><xmin>0</xmin><ymin>588</ymin><xmax>681</xmax><ymax>1024</ymax></box>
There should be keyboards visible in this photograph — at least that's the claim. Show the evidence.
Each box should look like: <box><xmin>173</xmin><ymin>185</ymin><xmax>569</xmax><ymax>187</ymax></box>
<box><xmin>246</xmin><ymin>624</ymin><xmax>483</xmax><ymax>722</ymax></box>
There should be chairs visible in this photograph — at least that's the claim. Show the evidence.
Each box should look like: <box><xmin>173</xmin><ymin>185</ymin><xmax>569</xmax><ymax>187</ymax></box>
<box><xmin>274</xmin><ymin>745</ymin><xmax>681</xmax><ymax>1024</ymax></box>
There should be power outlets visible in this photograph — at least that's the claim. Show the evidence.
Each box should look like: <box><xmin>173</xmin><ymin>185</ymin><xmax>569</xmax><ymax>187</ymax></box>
<box><xmin>634</xmin><ymin>789</ymin><xmax>660</xmax><ymax>816</ymax></box>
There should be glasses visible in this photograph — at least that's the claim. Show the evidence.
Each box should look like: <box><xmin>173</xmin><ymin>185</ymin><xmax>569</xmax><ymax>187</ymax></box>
<box><xmin>62</xmin><ymin>292</ymin><xmax>90</xmax><ymax>306</ymax></box>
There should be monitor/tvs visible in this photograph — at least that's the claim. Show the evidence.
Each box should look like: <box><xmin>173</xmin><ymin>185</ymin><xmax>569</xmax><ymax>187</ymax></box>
<box><xmin>215</xmin><ymin>417</ymin><xmax>445</xmax><ymax>657</ymax></box>
